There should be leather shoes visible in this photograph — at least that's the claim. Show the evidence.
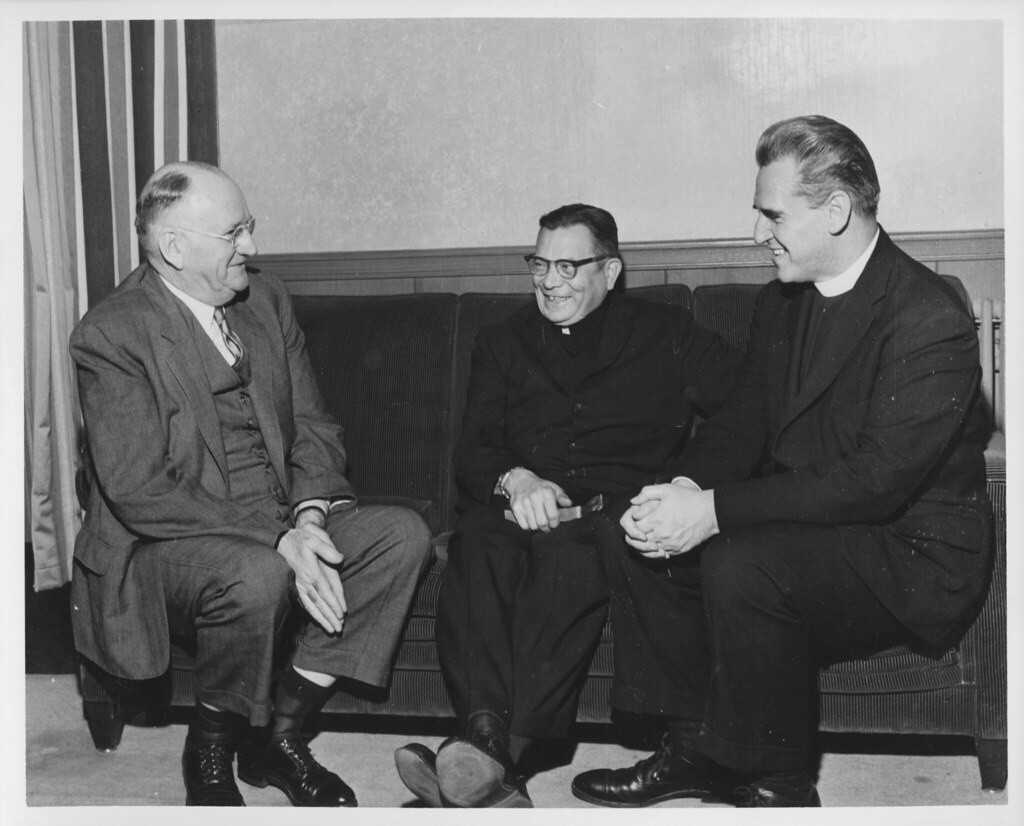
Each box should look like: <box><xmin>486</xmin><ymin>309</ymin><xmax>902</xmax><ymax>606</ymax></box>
<box><xmin>572</xmin><ymin>733</ymin><xmax>734</xmax><ymax>808</ymax></box>
<box><xmin>182</xmin><ymin>718</ymin><xmax>245</xmax><ymax>806</ymax></box>
<box><xmin>237</xmin><ymin>732</ymin><xmax>358</xmax><ymax>807</ymax></box>
<box><xmin>729</xmin><ymin>778</ymin><xmax>822</xmax><ymax>807</ymax></box>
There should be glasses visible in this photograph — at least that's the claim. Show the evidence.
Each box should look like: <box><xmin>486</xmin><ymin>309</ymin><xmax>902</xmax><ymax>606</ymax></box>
<box><xmin>524</xmin><ymin>251</ymin><xmax>608</xmax><ymax>279</ymax></box>
<box><xmin>169</xmin><ymin>216</ymin><xmax>256</xmax><ymax>249</ymax></box>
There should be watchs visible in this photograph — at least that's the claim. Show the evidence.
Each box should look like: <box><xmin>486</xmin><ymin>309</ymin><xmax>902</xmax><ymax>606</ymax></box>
<box><xmin>497</xmin><ymin>467</ymin><xmax>526</xmax><ymax>499</ymax></box>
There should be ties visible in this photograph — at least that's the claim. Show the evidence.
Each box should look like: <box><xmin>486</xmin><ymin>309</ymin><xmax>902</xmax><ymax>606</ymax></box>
<box><xmin>213</xmin><ymin>307</ymin><xmax>242</xmax><ymax>368</ymax></box>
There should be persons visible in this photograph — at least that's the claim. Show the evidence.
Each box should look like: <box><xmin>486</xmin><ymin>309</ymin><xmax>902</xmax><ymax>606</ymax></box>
<box><xmin>572</xmin><ymin>111</ymin><xmax>995</xmax><ymax>808</ymax></box>
<box><xmin>394</xmin><ymin>205</ymin><xmax>748</xmax><ymax>808</ymax></box>
<box><xmin>68</xmin><ymin>162</ymin><xmax>432</xmax><ymax>807</ymax></box>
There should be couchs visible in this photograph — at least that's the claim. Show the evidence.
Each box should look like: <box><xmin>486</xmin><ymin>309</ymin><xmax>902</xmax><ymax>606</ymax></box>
<box><xmin>74</xmin><ymin>272</ymin><xmax>1006</xmax><ymax>791</ymax></box>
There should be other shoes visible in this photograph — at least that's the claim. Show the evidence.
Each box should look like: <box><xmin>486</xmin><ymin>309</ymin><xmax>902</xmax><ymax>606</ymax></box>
<box><xmin>436</xmin><ymin>735</ymin><xmax>534</xmax><ymax>808</ymax></box>
<box><xmin>394</xmin><ymin>743</ymin><xmax>458</xmax><ymax>808</ymax></box>
